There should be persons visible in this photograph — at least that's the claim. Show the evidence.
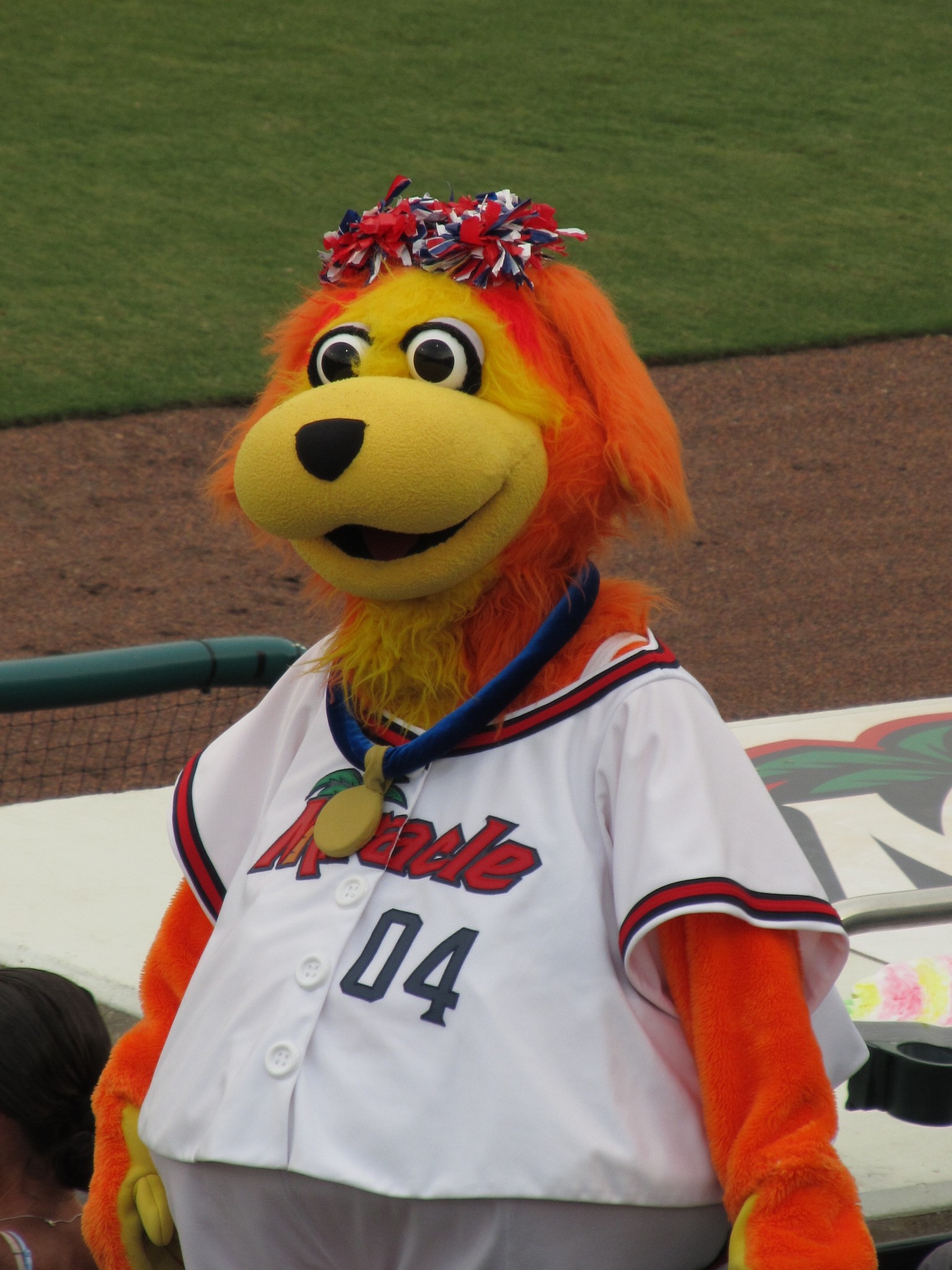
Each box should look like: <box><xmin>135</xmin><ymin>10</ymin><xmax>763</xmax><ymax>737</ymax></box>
<box><xmin>0</xmin><ymin>966</ymin><xmax>112</xmax><ymax>1270</ymax></box>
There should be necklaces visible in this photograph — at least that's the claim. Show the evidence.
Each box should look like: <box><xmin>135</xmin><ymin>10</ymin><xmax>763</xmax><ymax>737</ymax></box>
<box><xmin>0</xmin><ymin>1205</ymin><xmax>83</xmax><ymax>1228</ymax></box>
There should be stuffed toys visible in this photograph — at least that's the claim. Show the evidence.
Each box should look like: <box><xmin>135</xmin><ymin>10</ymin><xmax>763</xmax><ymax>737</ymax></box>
<box><xmin>73</xmin><ymin>153</ymin><xmax>884</xmax><ymax>1269</ymax></box>
